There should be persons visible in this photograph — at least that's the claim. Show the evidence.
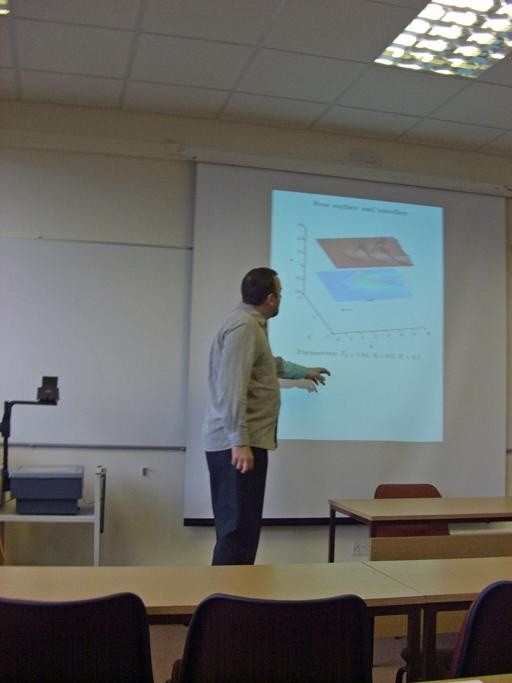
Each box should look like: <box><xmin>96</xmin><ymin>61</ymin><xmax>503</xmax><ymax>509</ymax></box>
<box><xmin>202</xmin><ymin>268</ymin><xmax>331</xmax><ymax>566</ymax></box>
<box><xmin>277</xmin><ymin>376</ymin><xmax>319</xmax><ymax>393</ymax></box>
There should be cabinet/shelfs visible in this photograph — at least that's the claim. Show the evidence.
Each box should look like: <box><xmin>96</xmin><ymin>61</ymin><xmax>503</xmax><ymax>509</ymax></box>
<box><xmin>0</xmin><ymin>470</ymin><xmax>102</xmax><ymax>567</ymax></box>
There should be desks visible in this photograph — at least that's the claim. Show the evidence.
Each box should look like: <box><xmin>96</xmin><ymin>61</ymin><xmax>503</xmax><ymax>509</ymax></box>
<box><xmin>1</xmin><ymin>560</ymin><xmax>425</xmax><ymax>667</ymax></box>
<box><xmin>326</xmin><ymin>498</ymin><xmax>511</xmax><ymax>561</ymax></box>
<box><xmin>357</xmin><ymin>554</ymin><xmax>512</xmax><ymax>665</ymax></box>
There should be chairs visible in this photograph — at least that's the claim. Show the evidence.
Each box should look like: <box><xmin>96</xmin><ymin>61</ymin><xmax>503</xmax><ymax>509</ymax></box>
<box><xmin>369</xmin><ymin>483</ymin><xmax>449</xmax><ymax>536</ymax></box>
<box><xmin>395</xmin><ymin>580</ymin><xmax>512</xmax><ymax>683</ymax></box>
<box><xmin>169</xmin><ymin>593</ymin><xmax>377</xmax><ymax>682</ymax></box>
<box><xmin>0</xmin><ymin>592</ymin><xmax>155</xmax><ymax>682</ymax></box>
